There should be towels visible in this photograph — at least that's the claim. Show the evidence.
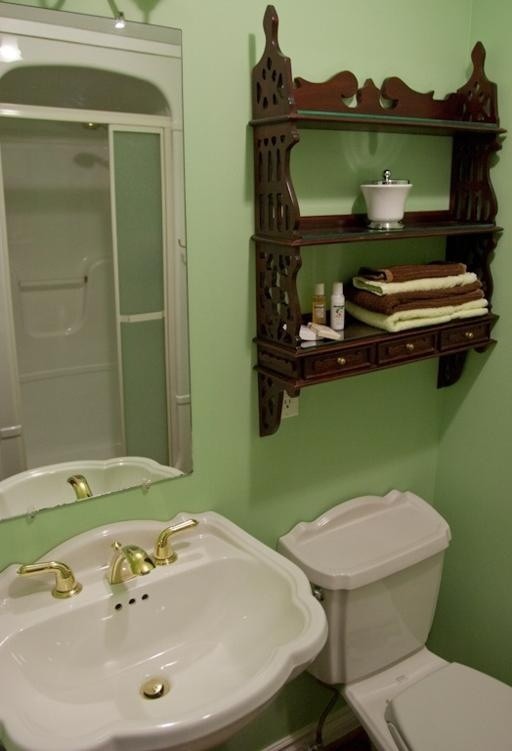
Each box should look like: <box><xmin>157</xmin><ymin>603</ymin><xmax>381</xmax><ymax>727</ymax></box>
<box><xmin>343</xmin><ymin>266</ymin><xmax>490</xmax><ymax>333</ymax></box>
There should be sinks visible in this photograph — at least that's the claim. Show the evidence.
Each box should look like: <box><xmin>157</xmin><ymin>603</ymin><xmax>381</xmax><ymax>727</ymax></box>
<box><xmin>0</xmin><ymin>455</ymin><xmax>185</xmax><ymax>521</ymax></box>
<box><xmin>0</xmin><ymin>510</ymin><xmax>330</xmax><ymax>751</ymax></box>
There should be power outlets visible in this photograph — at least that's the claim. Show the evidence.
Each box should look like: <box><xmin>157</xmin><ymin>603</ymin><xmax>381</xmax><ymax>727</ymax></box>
<box><xmin>282</xmin><ymin>390</ymin><xmax>300</xmax><ymax>417</ymax></box>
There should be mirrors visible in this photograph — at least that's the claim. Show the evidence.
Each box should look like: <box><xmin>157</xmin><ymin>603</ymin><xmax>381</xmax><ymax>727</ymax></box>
<box><xmin>1</xmin><ymin>0</ymin><xmax>193</xmax><ymax>520</ymax></box>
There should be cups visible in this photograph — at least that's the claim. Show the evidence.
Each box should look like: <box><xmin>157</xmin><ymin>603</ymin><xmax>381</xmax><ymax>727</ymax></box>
<box><xmin>358</xmin><ymin>183</ymin><xmax>415</xmax><ymax>232</ymax></box>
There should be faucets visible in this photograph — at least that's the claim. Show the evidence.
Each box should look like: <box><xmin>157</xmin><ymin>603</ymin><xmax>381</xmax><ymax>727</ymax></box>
<box><xmin>105</xmin><ymin>544</ymin><xmax>156</xmax><ymax>587</ymax></box>
<box><xmin>66</xmin><ymin>473</ymin><xmax>93</xmax><ymax>500</ymax></box>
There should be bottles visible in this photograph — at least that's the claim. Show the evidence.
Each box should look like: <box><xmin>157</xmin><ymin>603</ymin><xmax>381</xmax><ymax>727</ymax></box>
<box><xmin>311</xmin><ymin>283</ymin><xmax>325</xmax><ymax>326</ymax></box>
<box><xmin>330</xmin><ymin>283</ymin><xmax>344</xmax><ymax>331</ymax></box>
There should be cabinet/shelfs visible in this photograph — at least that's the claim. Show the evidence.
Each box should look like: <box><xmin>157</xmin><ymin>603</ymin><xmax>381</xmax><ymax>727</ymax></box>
<box><xmin>251</xmin><ymin>3</ymin><xmax>506</xmax><ymax>438</ymax></box>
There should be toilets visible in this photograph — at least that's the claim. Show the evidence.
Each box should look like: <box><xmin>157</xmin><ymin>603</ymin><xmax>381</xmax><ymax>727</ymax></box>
<box><xmin>278</xmin><ymin>489</ymin><xmax>511</xmax><ymax>751</ymax></box>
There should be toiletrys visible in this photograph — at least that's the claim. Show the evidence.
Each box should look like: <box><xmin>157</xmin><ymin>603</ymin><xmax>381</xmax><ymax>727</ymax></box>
<box><xmin>330</xmin><ymin>280</ymin><xmax>346</xmax><ymax>330</ymax></box>
<box><xmin>312</xmin><ymin>282</ymin><xmax>327</xmax><ymax>326</ymax></box>
